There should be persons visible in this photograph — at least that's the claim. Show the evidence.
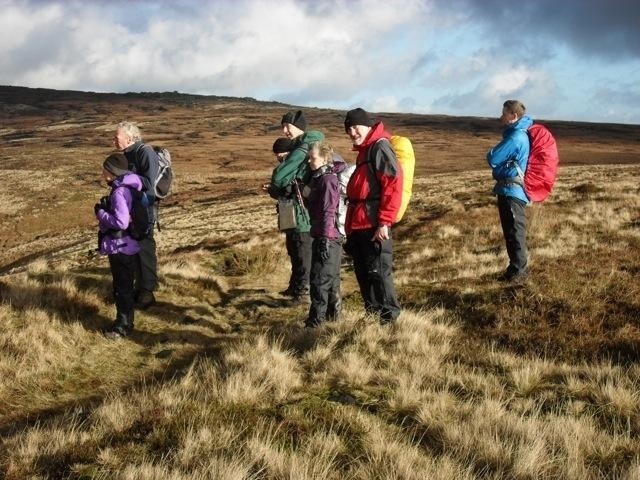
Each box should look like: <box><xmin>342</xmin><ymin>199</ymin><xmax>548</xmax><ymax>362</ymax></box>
<box><xmin>302</xmin><ymin>140</ymin><xmax>345</xmax><ymax>328</ymax></box>
<box><xmin>262</xmin><ymin>110</ymin><xmax>323</xmax><ymax>298</ymax></box>
<box><xmin>273</xmin><ymin>138</ymin><xmax>297</xmax><ymax>164</ymax></box>
<box><xmin>344</xmin><ymin>108</ymin><xmax>403</xmax><ymax>326</ymax></box>
<box><xmin>114</xmin><ymin>120</ymin><xmax>159</xmax><ymax>309</ymax></box>
<box><xmin>94</xmin><ymin>153</ymin><xmax>142</xmax><ymax>339</ymax></box>
<box><xmin>486</xmin><ymin>101</ymin><xmax>533</xmax><ymax>282</ymax></box>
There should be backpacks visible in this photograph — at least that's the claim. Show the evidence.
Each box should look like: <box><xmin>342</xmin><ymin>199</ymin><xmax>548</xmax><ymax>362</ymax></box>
<box><xmin>116</xmin><ymin>177</ymin><xmax>155</xmax><ymax>241</ymax></box>
<box><xmin>136</xmin><ymin>143</ymin><xmax>172</xmax><ymax>199</ymax></box>
<box><xmin>367</xmin><ymin>135</ymin><xmax>415</xmax><ymax>223</ymax></box>
<box><xmin>331</xmin><ymin>165</ymin><xmax>357</xmax><ymax>237</ymax></box>
<box><xmin>499</xmin><ymin>124</ymin><xmax>558</xmax><ymax>202</ymax></box>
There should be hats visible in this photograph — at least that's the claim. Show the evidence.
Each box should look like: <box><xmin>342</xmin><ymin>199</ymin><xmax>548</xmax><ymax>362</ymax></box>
<box><xmin>273</xmin><ymin>138</ymin><xmax>293</xmax><ymax>152</ymax></box>
<box><xmin>104</xmin><ymin>154</ymin><xmax>128</xmax><ymax>177</ymax></box>
<box><xmin>282</xmin><ymin>109</ymin><xmax>306</xmax><ymax>131</ymax></box>
<box><xmin>344</xmin><ymin>108</ymin><xmax>371</xmax><ymax>132</ymax></box>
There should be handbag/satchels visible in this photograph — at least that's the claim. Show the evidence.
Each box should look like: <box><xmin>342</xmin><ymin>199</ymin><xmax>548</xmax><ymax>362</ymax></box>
<box><xmin>279</xmin><ymin>202</ymin><xmax>299</xmax><ymax>231</ymax></box>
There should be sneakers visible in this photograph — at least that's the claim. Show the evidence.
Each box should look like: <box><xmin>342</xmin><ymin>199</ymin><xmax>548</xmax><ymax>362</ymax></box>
<box><xmin>137</xmin><ymin>290</ymin><xmax>154</xmax><ymax>309</ymax></box>
<box><xmin>104</xmin><ymin>325</ymin><xmax>133</xmax><ymax>339</ymax></box>
<box><xmin>497</xmin><ymin>262</ymin><xmax>526</xmax><ymax>280</ymax></box>
<box><xmin>278</xmin><ymin>286</ymin><xmax>308</xmax><ymax>296</ymax></box>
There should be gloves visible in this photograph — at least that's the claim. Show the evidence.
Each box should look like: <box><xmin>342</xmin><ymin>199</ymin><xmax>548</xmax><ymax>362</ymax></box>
<box><xmin>319</xmin><ymin>237</ymin><xmax>329</xmax><ymax>261</ymax></box>
<box><xmin>94</xmin><ymin>195</ymin><xmax>110</xmax><ymax>214</ymax></box>
